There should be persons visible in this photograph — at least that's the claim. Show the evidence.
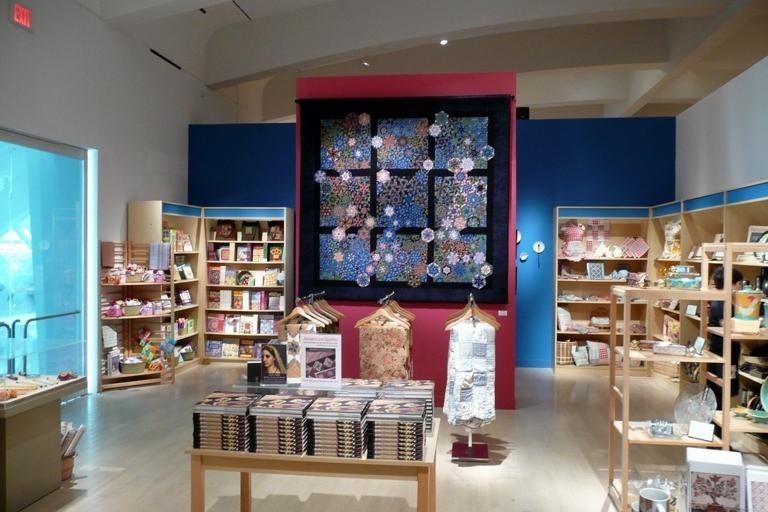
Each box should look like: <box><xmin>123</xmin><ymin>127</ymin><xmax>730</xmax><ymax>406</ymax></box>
<box><xmin>261</xmin><ymin>345</ymin><xmax>285</xmax><ymax>375</ymax></box>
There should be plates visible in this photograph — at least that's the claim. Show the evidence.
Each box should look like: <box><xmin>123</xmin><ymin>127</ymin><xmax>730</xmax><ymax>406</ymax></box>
<box><xmin>673</xmin><ymin>384</ymin><xmax>718</xmax><ymax>434</ymax></box>
<box><xmin>733</xmin><ymin>376</ymin><xmax>768</xmax><ymax>424</ymax></box>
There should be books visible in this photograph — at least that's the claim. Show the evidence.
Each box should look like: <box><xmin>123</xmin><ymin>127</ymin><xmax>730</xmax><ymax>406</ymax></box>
<box><xmin>254</xmin><ymin>342</ymin><xmax>287</xmax><ymax>386</ymax></box>
<box><xmin>191</xmin><ymin>376</ymin><xmax>434</xmax><ymax>461</ymax></box>
<box><xmin>244</xmin><ymin>361</ymin><xmax>260</xmax><ymax>382</ymax></box>
<box><xmin>205</xmin><ymin>219</ymin><xmax>284</xmax><ymax>359</ymax></box>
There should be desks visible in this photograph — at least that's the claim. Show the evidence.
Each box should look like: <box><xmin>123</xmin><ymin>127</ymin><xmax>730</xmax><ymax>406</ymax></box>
<box><xmin>193</xmin><ymin>379</ymin><xmax>442</xmax><ymax>510</ymax></box>
<box><xmin>0</xmin><ymin>373</ymin><xmax>89</xmax><ymax>510</ymax></box>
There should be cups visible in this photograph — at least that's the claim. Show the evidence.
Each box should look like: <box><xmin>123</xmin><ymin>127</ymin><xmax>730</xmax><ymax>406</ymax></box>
<box><xmin>639</xmin><ymin>487</ymin><xmax>670</xmax><ymax>512</ymax></box>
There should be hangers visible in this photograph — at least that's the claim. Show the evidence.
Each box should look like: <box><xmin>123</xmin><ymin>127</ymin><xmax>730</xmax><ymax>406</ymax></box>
<box><xmin>443</xmin><ymin>300</ymin><xmax>502</xmax><ymax>331</ymax></box>
<box><xmin>272</xmin><ymin>293</ymin><xmax>344</xmax><ymax>328</ymax></box>
<box><xmin>353</xmin><ymin>297</ymin><xmax>418</xmax><ymax>330</ymax></box>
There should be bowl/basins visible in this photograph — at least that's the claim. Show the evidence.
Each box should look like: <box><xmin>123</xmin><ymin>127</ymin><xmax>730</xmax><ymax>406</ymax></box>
<box><xmin>637</xmin><ymin>340</ymin><xmax>659</xmax><ymax>351</ymax></box>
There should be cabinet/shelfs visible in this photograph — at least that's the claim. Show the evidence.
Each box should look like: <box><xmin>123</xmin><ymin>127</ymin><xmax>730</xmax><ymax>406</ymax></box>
<box><xmin>608</xmin><ymin>285</ymin><xmax>731</xmax><ymax>512</ymax></box>
<box><xmin>204</xmin><ymin>204</ymin><xmax>295</xmax><ymax>364</ymax></box>
<box><xmin>126</xmin><ymin>200</ymin><xmax>203</xmax><ymax>374</ymax></box>
<box><xmin>554</xmin><ymin>205</ymin><xmax>655</xmax><ymax>379</ymax></box>
<box><xmin>96</xmin><ymin>240</ymin><xmax>178</xmax><ymax>391</ymax></box>
<box><xmin>654</xmin><ymin>182</ymin><xmax>766</xmax><ymax>411</ymax></box>
